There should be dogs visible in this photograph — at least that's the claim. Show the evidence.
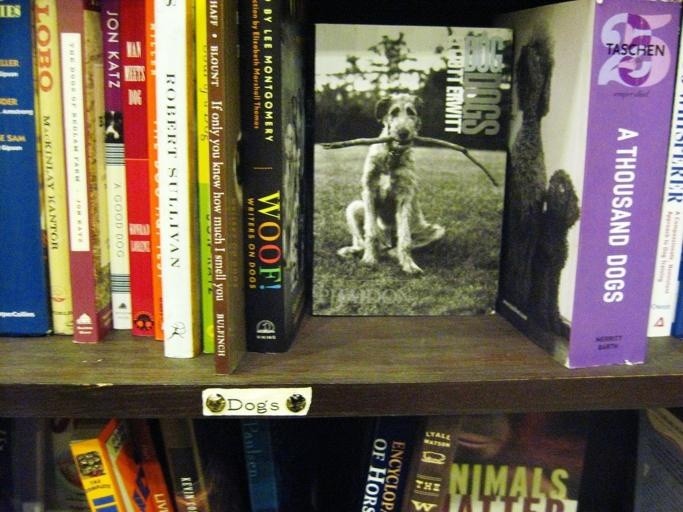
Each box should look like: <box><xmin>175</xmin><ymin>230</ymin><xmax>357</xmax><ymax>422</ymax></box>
<box><xmin>503</xmin><ymin>36</ymin><xmax>554</xmax><ymax>315</ymax></box>
<box><xmin>526</xmin><ymin>169</ymin><xmax>579</xmax><ymax>349</ymax></box>
<box><xmin>102</xmin><ymin>109</ymin><xmax>124</xmax><ymax>143</ymax></box>
<box><xmin>336</xmin><ymin>92</ymin><xmax>446</xmax><ymax>278</ymax></box>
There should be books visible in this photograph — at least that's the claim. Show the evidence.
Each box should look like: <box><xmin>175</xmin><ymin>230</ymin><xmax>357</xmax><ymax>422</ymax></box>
<box><xmin>1</xmin><ymin>0</ymin><xmax>682</xmax><ymax>371</ymax></box>
<box><xmin>1</xmin><ymin>408</ymin><xmax>682</xmax><ymax>511</ymax></box>
